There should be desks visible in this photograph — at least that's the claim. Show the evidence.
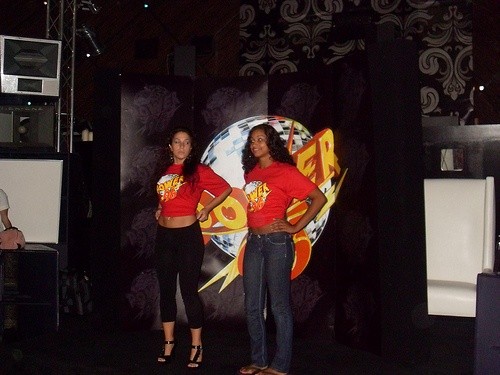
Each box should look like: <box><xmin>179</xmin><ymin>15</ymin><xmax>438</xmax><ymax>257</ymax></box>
<box><xmin>0</xmin><ymin>244</ymin><xmax>60</xmax><ymax>342</ymax></box>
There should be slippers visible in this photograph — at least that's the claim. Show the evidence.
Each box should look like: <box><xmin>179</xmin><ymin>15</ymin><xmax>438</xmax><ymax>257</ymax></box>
<box><xmin>255</xmin><ymin>366</ymin><xmax>289</xmax><ymax>375</ymax></box>
<box><xmin>236</xmin><ymin>365</ymin><xmax>269</xmax><ymax>375</ymax></box>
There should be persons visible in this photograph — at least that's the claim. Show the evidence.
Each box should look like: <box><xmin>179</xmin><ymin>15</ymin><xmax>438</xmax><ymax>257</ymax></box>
<box><xmin>0</xmin><ymin>189</ymin><xmax>13</xmax><ymax>232</ymax></box>
<box><xmin>155</xmin><ymin>129</ymin><xmax>232</xmax><ymax>367</ymax></box>
<box><xmin>240</xmin><ymin>124</ymin><xmax>327</xmax><ymax>375</ymax></box>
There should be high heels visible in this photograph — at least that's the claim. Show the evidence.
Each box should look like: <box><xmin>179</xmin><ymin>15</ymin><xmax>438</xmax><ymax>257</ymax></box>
<box><xmin>157</xmin><ymin>340</ymin><xmax>177</xmax><ymax>363</ymax></box>
<box><xmin>186</xmin><ymin>344</ymin><xmax>205</xmax><ymax>370</ymax></box>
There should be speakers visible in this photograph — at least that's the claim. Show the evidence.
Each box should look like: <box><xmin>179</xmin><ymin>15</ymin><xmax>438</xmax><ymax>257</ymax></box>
<box><xmin>0</xmin><ymin>35</ymin><xmax>62</xmax><ymax>97</ymax></box>
<box><xmin>473</xmin><ymin>273</ymin><xmax>500</xmax><ymax>375</ymax></box>
<box><xmin>0</xmin><ymin>105</ymin><xmax>56</xmax><ymax>148</ymax></box>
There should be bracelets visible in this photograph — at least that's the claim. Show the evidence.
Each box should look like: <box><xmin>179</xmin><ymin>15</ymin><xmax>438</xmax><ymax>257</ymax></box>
<box><xmin>157</xmin><ymin>207</ymin><xmax>162</xmax><ymax>210</ymax></box>
